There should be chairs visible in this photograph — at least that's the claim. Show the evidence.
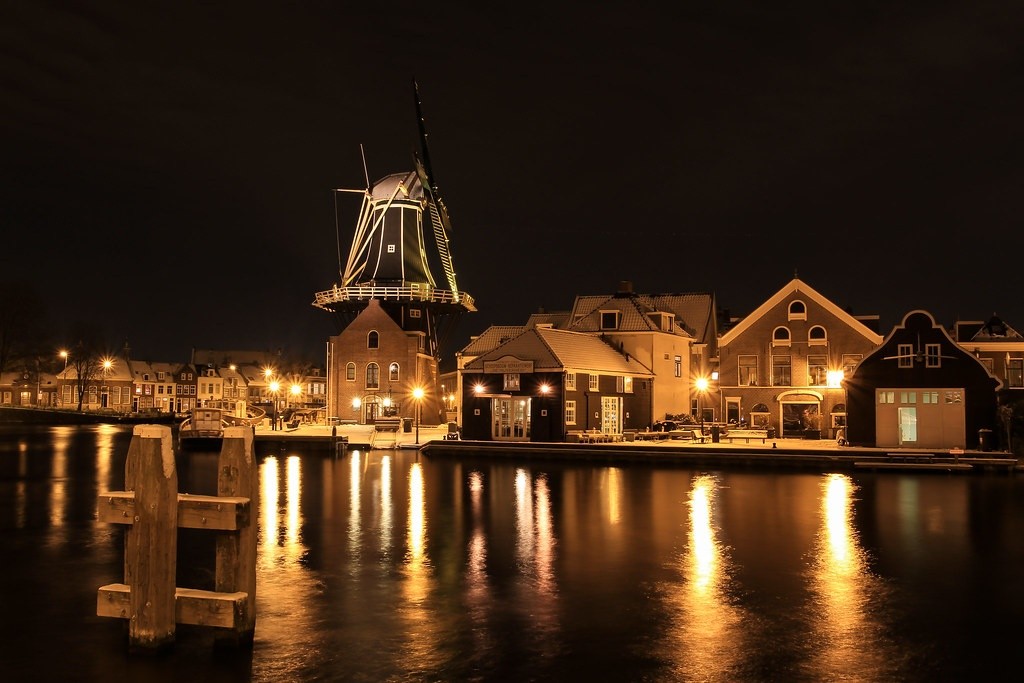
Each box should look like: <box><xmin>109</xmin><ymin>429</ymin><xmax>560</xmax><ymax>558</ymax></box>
<box><xmin>287</xmin><ymin>419</ymin><xmax>300</xmax><ymax>428</ymax></box>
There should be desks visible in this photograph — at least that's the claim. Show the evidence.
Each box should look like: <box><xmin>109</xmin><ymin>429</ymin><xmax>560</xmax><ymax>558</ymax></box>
<box><xmin>638</xmin><ymin>431</ymin><xmax>668</xmax><ymax>440</ymax></box>
<box><xmin>669</xmin><ymin>430</ymin><xmax>691</xmax><ymax>440</ymax></box>
<box><xmin>677</xmin><ymin>424</ymin><xmax>710</xmax><ymax>431</ymax></box>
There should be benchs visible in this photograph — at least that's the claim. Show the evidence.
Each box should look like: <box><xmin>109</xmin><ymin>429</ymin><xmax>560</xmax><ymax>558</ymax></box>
<box><xmin>566</xmin><ymin>429</ymin><xmax>637</xmax><ymax>443</ymax></box>
<box><xmin>374</xmin><ymin>419</ymin><xmax>401</xmax><ymax>433</ymax></box>
<box><xmin>691</xmin><ymin>430</ymin><xmax>710</xmax><ymax>442</ymax></box>
<box><xmin>727</xmin><ymin>429</ymin><xmax>768</xmax><ymax>443</ymax></box>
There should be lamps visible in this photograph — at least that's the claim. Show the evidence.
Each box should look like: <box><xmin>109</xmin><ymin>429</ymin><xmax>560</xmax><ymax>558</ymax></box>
<box><xmin>915</xmin><ymin>350</ymin><xmax>923</xmax><ymax>363</ymax></box>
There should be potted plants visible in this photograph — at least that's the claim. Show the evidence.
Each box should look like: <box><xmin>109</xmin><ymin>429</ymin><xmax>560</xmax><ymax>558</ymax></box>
<box><xmin>804</xmin><ymin>408</ymin><xmax>824</xmax><ymax>439</ymax></box>
